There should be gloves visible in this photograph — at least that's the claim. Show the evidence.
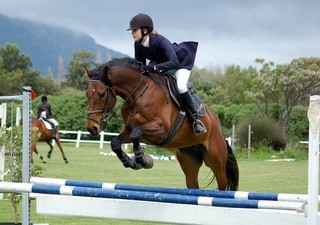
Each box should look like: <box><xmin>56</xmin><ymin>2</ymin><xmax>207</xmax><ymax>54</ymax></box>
<box><xmin>137</xmin><ymin>62</ymin><xmax>154</xmax><ymax>75</ymax></box>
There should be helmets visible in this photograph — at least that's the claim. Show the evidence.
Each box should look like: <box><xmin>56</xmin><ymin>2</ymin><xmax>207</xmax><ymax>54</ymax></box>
<box><xmin>126</xmin><ymin>13</ymin><xmax>153</xmax><ymax>31</ymax></box>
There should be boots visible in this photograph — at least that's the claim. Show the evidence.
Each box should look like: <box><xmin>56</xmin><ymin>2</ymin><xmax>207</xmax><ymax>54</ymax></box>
<box><xmin>52</xmin><ymin>125</ymin><xmax>58</xmax><ymax>137</ymax></box>
<box><xmin>179</xmin><ymin>89</ymin><xmax>205</xmax><ymax>134</ymax></box>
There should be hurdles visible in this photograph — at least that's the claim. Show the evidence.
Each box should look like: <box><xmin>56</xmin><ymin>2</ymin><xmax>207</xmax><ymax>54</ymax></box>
<box><xmin>0</xmin><ymin>86</ymin><xmax>320</xmax><ymax>225</ymax></box>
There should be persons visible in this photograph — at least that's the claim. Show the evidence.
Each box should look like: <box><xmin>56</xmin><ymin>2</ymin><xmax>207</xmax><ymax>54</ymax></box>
<box><xmin>126</xmin><ymin>13</ymin><xmax>205</xmax><ymax>134</ymax></box>
<box><xmin>37</xmin><ymin>95</ymin><xmax>57</xmax><ymax>139</ymax></box>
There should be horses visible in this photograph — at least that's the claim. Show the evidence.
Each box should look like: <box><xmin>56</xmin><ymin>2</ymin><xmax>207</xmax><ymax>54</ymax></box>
<box><xmin>21</xmin><ymin>117</ymin><xmax>69</xmax><ymax>165</ymax></box>
<box><xmin>82</xmin><ymin>56</ymin><xmax>240</xmax><ymax>192</ymax></box>
